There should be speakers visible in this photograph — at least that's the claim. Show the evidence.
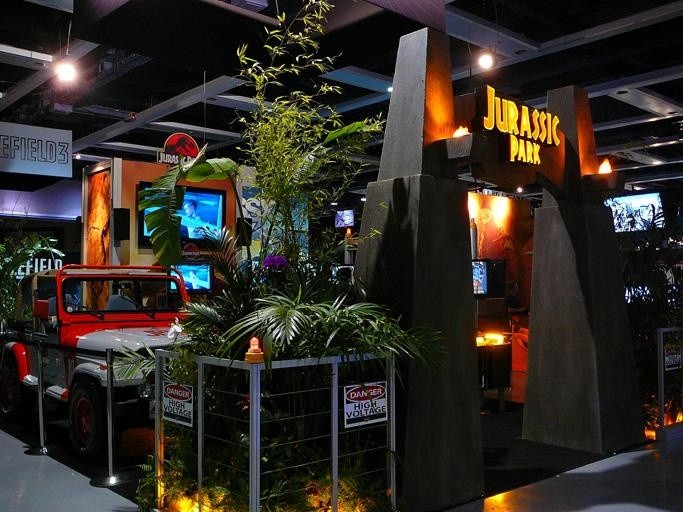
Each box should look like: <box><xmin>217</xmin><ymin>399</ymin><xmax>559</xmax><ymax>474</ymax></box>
<box><xmin>114</xmin><ymin>207</ymin><xmax>130</xmax><ymax>240</ymax></box>
<box><xmin>237</xmin><ymin>217</ymin><xmax>252</xmax><ymax>246</ymax></box>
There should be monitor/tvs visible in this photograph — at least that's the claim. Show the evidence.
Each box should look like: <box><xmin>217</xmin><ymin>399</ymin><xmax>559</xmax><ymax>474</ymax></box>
<box><xmin>603</xmin><ymin>189</ymin><xmax>668</xmax><ymax>235</ymax></box>
<box><xmin>333</xmin><ymin>207</ymin><xmax>355</xmax><ymax>229</ymax></box>
<box><xmin>138</xmin><ymin>180</ymin><xmax>226</xmax><ymax>249</ymax></box>
<box><xmin>472</xmin><ymin>258</ymin><xmax>490</xmax><ymax>299</ymax></box>
<box><xmin>168</xmin><ymin>260</ymin><xmax>214</xmax><ymax>291</ymax></box>
<box><xmin>489</xmin><ymin>258</ymin><xmax>506</xmax><ymax>299</ymax></box>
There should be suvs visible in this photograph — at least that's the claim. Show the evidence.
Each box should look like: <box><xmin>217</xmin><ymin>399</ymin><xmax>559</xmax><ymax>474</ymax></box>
<box><xmin>1</xmin><ymin>264</ymin><xmax>231</xmax><ymax>466</ymax></box>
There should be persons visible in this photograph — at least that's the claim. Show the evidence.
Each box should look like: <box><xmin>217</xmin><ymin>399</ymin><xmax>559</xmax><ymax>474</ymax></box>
<box><xmin>337</xmin><ymin>213</ymin><xmax>353</xmax><ymax>227</ymax></box>
<box><xmin>182</xmin><ymin>199</ymin><xmax>200</xmax><ymax>221</ymax></box>
<box><xmin>188</xmin><ymin>270</ymin><xmax>200</xmax><ymax>280</ymax></box>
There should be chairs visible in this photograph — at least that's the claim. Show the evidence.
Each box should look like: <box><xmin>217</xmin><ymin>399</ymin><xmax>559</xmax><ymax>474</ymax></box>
<box><xmin>47</xmin><ymin>293</ymin><xmax>76</xmax><ymax>327</ymax></box>
<box><xmin>106</xmin><ymin>295</ymin><xmax>137</xmax><ymax>310</ymax></box>
